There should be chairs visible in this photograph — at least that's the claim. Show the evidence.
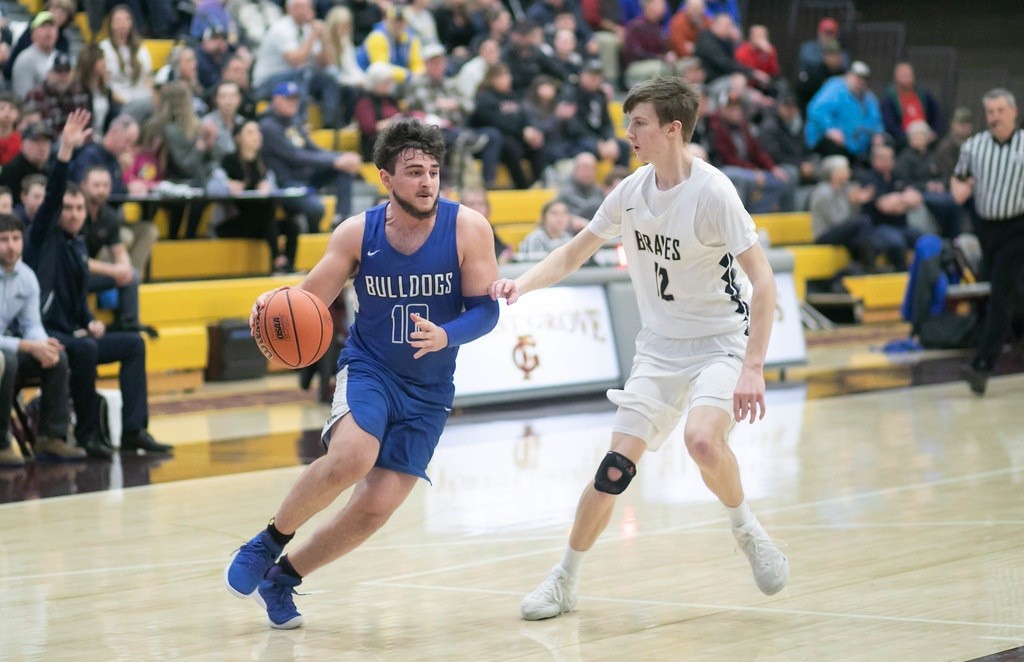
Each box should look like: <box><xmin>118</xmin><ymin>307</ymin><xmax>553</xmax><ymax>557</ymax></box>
<box><xmin>935</xmin><ymin>232</ymin><xmax>988</xmax><ymax>333</ymax></box>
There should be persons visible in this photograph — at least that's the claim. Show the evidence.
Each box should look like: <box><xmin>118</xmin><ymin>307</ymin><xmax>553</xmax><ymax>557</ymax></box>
<box><xmin>207</xmin><ymin>119</ymin><xmax>311</xmax><ymax>277</ymax></box>
<box><xmin>224</xmin><ymin>115</ymin><xmax>499</xmax><ymax>630</ymax></box>
<box><xmin>0</xmin><ymin>0</ymin><xmax>799</xmax><ymax>214</ymax></box>
<box><xmin>558</xmin><ymin>152</ymin><xmax>605</xmax><ymax>222</ymax></box>
<box><xmin>797</xmin><ymin>16</ymin><xmax>1024</xmax><ymax>395</ymax></box>
<box><xmin>1</xmin><ymin>108</ymin><xmax>175</xmax><ymax>468</ymax></box>
<box><xmin>488</xmin><ymin>74</ymin><xmax>790</xmax><ymax>620</ymax></box>
<box><xmin>519</xmin><ymin>200</ymin><xmax>593</xmax><ymax>265</ymax></box>
<box><xmin>458</xmin><ymin>186</ymin><xmax>510</xmax><ymax>265</ymax></box>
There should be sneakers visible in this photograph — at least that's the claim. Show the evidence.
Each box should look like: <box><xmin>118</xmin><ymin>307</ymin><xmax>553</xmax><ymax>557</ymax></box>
<box><xmin>520</xmin><ymin>564</ymin><xmax>579</xmax><ymax>620</ymax></box>
<box><xmin>225</xmin><ymin>528</ymin><xmax>283</xmax><ymax>599</ymax></box>
<box><xmin>253</xmin><ymin>564</ymin><xmax>309</xmax><ymax>629</ymax></box>
<box><xmin>733</xmin><ymin>517</ymin><xmax>787</xmax><ymax>595</ymax></box>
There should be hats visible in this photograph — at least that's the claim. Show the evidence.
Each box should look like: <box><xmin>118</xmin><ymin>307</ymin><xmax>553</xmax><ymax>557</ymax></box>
<box><xmin>30</xmin><ymin>10</ymin><xmax>55</xmax><ymax>30</ymax></box>
<box><xmin>22</xmin><ymin>124</ymin><xmax>55</xmax><ymax>143</ymax></box>
<box><xmin>272</xmin><ymin>82</ymin><xmax>301</xmax><ymax>97</ymax></box>
<box><xmin>47</xmin><ymin>54</ymin><xmax>73</xmax><ymax>72</ymax></box>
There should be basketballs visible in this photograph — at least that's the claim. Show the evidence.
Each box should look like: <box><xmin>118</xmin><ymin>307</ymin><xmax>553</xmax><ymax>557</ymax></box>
<box><xmin>252</xmin><ymin>288</ymin><xmax>333</xmax><ymax>369</ymax></box>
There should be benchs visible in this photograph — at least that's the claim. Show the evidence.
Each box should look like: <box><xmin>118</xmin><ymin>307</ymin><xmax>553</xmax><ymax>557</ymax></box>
<box><xmin>23</xmin><ymin>0</ymin><xmax>973</xmax><ymax>377</ymax></box>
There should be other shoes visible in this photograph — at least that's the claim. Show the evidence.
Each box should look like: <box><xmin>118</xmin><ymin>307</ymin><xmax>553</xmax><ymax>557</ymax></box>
<box><xmin>959</xmin><ymin>366</ymin><xmax>987</xmax><ymax>391</ymax></box>
<box><xmin>0</xmin><ymin>445</ymin><xmax>25</xmax><ymax>466</ymax></box>
<box><xmin>35</xmin><ymin>435</ymin><xmax>87</xmax><ymax>460</ymax></box>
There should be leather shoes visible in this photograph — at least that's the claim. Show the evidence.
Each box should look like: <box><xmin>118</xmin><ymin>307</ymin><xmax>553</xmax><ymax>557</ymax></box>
<box><xmin>77</xmin><ymin>439</ymin><xmax>112</xmax><ymax>458</ymax></box>
<box><xmin>121</xmin><ymin>431</ymin><xmax>173</xmax><ymax>451</ymax></box>
